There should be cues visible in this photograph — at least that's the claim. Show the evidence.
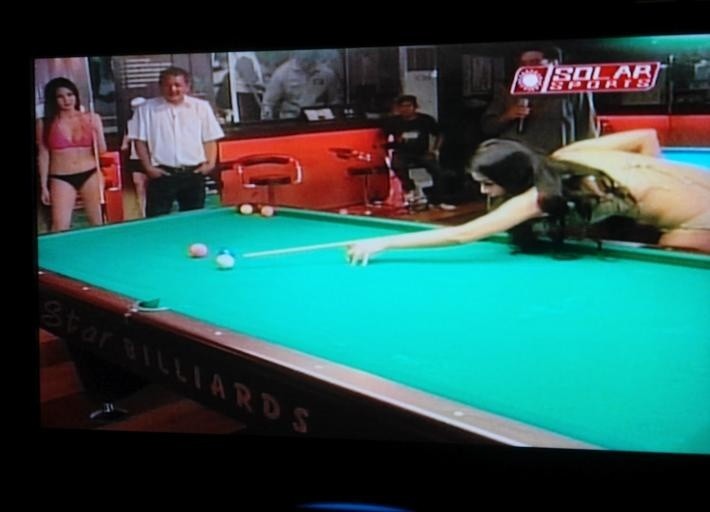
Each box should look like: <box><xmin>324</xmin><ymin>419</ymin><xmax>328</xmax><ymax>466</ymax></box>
<box><xmin>90</xmin><ymin>102</ymin><xmax>107</xmax><ymax>224</ymax></box>
<box><xmin>242</xmin><ymin>224</ymin><xmax>385</xmax><ymax>260</ymax></box>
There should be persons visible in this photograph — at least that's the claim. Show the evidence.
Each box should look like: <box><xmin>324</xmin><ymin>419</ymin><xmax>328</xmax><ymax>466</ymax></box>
<box><xmin>35</xmin><ymin>77</ymin><xmax>107</xmax><ymax>232</ymax></box>
<box><xmin>481</xmin><ymin>45</ymin><xmax>600</xmax><ymax>155</ymax></box>
<box><xmin>381</xmin><ymin>94</ymin><xmax>458</xmax><ymax>210</ymax></box>
<box><xmin>346</xmin><ymin>128</ymin><xmax>710</xmax><ymax>265</ymax></box>
<box><xmin>260</xmin><ymin>50</ymin><xmax>345</xmax><ymax>121</ymax></box>
<box><xmin>121</xmin><ymin>67</ymin><xmax>224</xmax><ymax>217</ymax></box>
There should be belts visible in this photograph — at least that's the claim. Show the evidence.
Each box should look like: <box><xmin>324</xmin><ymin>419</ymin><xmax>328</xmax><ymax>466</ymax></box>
<box><xmin>166</xmin><ymin>167</ymin><xmax>193</xmax><ymax>174</ymax></box>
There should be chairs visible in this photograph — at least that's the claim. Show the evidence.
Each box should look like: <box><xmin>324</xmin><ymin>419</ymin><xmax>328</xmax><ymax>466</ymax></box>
<box><xmin>335</xmin><ymin>147</ymin><xmax>391</xmax><ymax>209</ymax></box>
<box><xmin>234</xmin><ymin>152</ymin><xmax>305</xmax><ymax>204</ymax></box>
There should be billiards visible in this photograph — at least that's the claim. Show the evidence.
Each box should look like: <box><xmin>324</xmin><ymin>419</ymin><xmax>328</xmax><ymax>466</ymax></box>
<box><xmin>215</xmin><ymin>255</ymin><xmax>236</xmax><ymax>271</ymax></box>
<box><xmin>217</xmin><ymin>248</ymin><xmax>234</xmax><ymax>257</ymax></box>
<box><xmin>239</xmin><ymin>203</ymin><xmax>254</xmax><ymax>215</ymax></box>
<box><xmin>260</xmin><ymin>205</ymin><xmax>275</xmax><ymax>218</ymax></box>
<box><xmin>189</xmin><ymin>243</ymin><xmax>208</xmax><ymax>259</ymax></box>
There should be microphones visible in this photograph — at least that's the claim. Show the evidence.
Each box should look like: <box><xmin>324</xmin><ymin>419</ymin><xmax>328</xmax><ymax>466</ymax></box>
<box><xmin>517</xmin><ymin>98</ymin><xmax>529</xmax><ymax>134</ymax></box>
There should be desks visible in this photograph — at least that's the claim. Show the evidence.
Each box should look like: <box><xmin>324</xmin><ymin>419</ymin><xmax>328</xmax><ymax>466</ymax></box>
<box><xmin>38</xmin><ymin>199</ymin><xmax>710</xmax><ymax>457</ymax></box>
